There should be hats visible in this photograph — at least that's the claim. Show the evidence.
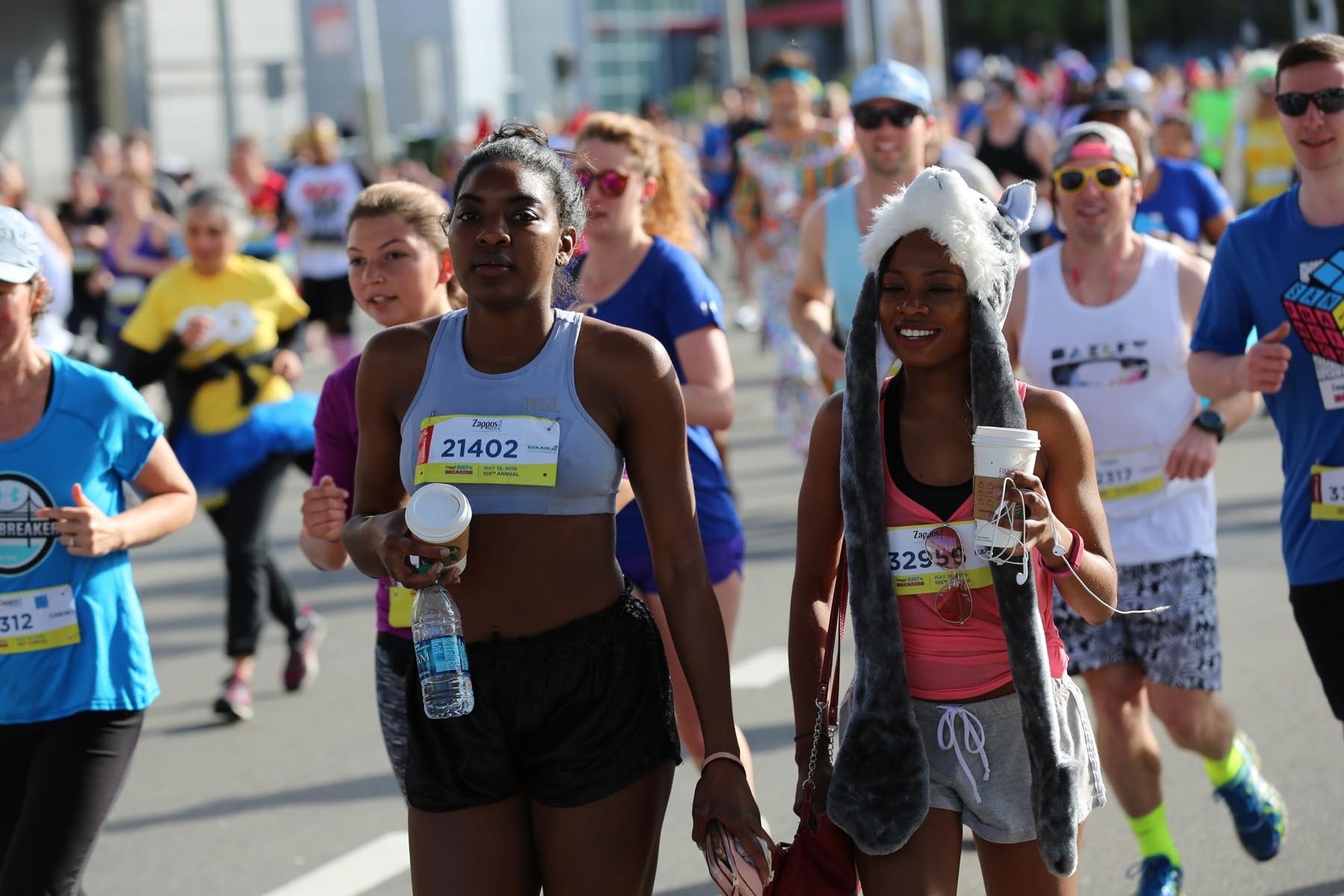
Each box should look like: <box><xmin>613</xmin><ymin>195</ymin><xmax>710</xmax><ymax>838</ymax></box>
<box><xmin>1091</xmin><ymin>83</ymin><xmax>1134</xmax><ymax>112</ymax></box>
<box><xmin>850</xmin><ymin>58</ymin><xmax>930</xmax><ymax>113</ymax></box>
<box><xmin>0</xmin><ymin>206</ymin><xmax>41</xmax><ymax>284</ymax></box>
<box><xmin>1054</xmin><ymin>121</ymin><xmax>1138</xmax><ymax>181</ymax></box>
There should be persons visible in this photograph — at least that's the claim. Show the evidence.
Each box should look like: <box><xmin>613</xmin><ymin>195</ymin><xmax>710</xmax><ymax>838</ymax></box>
<box><xmin>788</xmin><ymin>59</ymin><xmax>1285</xmax><ymax>896</ymax></box>
<box><xmin>0</xmin><ymin>19</ymin><xmax>1296</xmax><ymax>385</ymax></box>
<box><xmin>299</xmin><ymin>120</ymin><xmax>781</xmax><ymax>896</ymax></box>
<box><xmin>1186</xmin><ymin>35</ymin><xmax>1344</xmax><ymax>723</ymax></box>
<box><xmin>111</xmin><ymin>178</ymin><xmax>321</xmax><ymax>726</ymax></box>
<box><xmin>0</xmin><ymin>204</ymin><xmax>197</xmax><ymax>896</ymax></box>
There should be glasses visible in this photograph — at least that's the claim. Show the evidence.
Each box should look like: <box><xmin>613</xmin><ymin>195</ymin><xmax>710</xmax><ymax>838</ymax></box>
<box><xmin>854</xmin><ymin>107</ymin><xmax>921</xmax><ymax>129</ymax></box>
<box><xmin>1054</xmin><ymin>160</ymin><xmax>1134</xmax><ymax>194</ymax></box>
<box><xmin>574</xmin><ymin>164</ymin><xmax>648</xmax><ymax>198</ymax></box>
<box><xmin>1275</xmin><ymin>86</ymin><xmax>1344</xmax><ymax>117</ymax></box>
<box><xmin>926</xmin><ymin>522</ymin><xmax>973</xmax><ymax>625</ymax></box>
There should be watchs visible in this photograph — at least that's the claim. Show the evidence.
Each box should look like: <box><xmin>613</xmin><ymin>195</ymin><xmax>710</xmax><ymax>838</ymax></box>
<box><xmin>1191</xmin><ymin>408</ymin><xmax>1226</xmax><ymax>442</ymax></box>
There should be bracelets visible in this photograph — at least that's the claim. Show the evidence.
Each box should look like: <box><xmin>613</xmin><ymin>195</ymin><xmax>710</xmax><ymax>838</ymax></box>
<box><xmin>1037</xmin><ymin>527</ymin><xmax>1085</xmax><ymax>579</ymax></box>
<box><xmin>792</xmin><ymin>732</ymin><xmax>831</xmax><ymax>746</ymax></box>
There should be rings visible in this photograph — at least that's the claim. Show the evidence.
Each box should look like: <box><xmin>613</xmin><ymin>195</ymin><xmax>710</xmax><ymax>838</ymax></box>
<box><xmin>68</xmin><ymin>537</ymin><xmax>75</xmax><ymax>547</ymax></box>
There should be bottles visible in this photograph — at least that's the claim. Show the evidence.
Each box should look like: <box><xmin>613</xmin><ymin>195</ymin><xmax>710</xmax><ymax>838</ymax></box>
<box><xmin>413</xmin><ymin>562</ymin><xmax>475</xmax><ymax>718</ymax></box>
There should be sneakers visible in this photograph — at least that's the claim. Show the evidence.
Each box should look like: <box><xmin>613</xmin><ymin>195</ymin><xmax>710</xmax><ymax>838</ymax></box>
<box><xmin>1126</xmin><ymin>855</ymin><xmax>1184</xmax><ymax>896</ymax></box>
<box><xmin>285</xmin><ymin>608</ymin><xmax>326</xmax><ymax>696</ymax></box>
<box><xmin>214</xmin><ymin>676</ymin><xmax>256</xmax><ymax>717</ymax></box>
<box><xmin>1212</xmin><ymin>731</ymin><xmax>1286</xmax><ymax>862</ymax></box>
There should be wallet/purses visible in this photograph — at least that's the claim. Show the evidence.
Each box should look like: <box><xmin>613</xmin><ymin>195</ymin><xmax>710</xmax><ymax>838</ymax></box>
<box><xmin>703</xmin><ymin>753</ymin><xmax>765</xmax><ymax>896</ymax></box>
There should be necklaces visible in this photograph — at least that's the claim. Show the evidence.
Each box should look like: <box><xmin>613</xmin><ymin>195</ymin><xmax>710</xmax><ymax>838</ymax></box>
<box><xmin>1065</xmin><ymin>231</ymin><xmax>1124</xmax><ymax>307</ymax></box>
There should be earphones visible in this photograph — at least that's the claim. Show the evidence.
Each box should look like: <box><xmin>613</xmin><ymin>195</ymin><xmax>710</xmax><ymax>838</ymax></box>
<box><xmin>1052</xmin><ymin>530</ymin><xmax>1065</xmax><ymax>557</ymax></box>
<box><xmin>1016</xmin><ymin>555</ymin><xmax>1028</xmax><ymax>585</ymax></box>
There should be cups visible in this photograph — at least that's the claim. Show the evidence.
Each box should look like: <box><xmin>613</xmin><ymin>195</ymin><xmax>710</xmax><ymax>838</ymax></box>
<box><xmin>969</xmin><ymin>426</ymin><xmax>1043</xmax><ymax>548</ymax></box>
<box><xmin>403</xmin><ymin>482</ymin><xmax>474</xmax><ymax>580</ymax></box>
